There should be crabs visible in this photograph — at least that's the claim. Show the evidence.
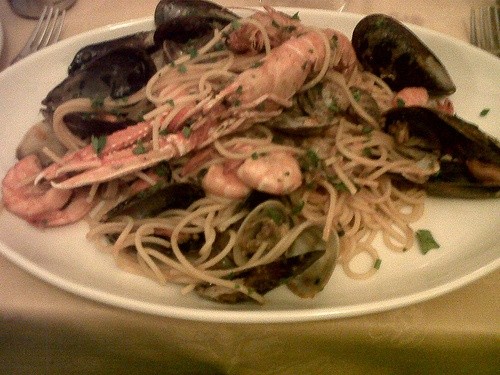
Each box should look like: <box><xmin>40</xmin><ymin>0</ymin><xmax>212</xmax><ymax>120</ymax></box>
<box><xmin>32</xmin><ymin>105</ymin><xmax>254</xmax><ymax>192</ymax></box>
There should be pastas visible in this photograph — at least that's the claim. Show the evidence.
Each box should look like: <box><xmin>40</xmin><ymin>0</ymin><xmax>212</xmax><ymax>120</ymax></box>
<box><xmin>2</xmin><ymin>1</ymin><xmax>500</xmax><ymax>305</ymax></box>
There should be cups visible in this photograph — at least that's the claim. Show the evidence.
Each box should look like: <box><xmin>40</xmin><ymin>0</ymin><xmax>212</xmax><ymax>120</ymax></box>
<box><xmin>9</xmin><ymin>0</ymin><xmax>76</xmax><ymax>18</ymax></box>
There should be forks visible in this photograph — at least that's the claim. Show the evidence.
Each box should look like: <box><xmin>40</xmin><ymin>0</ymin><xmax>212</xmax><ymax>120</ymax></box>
<box><xmin>12</xmin><ymin>5</ymin><xmax>66</xmax><ymax>64</ymax></box>
<box><xmin>470</xmin><ymin>4</ymin><xmax>500</xmax><ymax>59</ymax></box>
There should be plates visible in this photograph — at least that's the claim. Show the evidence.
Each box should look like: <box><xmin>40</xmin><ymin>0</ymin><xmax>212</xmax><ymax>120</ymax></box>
<box><xmin>0</xmin><ymin>7</ymin><xmax>500</xmax><ymax>323</ymax></box>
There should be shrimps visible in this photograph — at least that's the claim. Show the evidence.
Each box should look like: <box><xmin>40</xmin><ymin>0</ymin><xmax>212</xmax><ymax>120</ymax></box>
<box><xmin>200</xmin><ymin>151</ymin><xmax>304</xmax><ymax>202</ymax></box>
<box><xmin>226</xmin><ymin>5</ymin><xmax>306</xmax><ymax>52</ymax></box>
<box><xmin>1</xmin><ymin>153</ymin><xmax>101</xmax><ymax>226</ymax></box>
<box><xmin>234</xmin><ymin>28</ymin><xmax>363</xmax><ymax>116</ymax></box>
<box><xmin>393</xmin><ymin>87</ymin><xmax>454</xmax><ymax>116</ymax></box>
